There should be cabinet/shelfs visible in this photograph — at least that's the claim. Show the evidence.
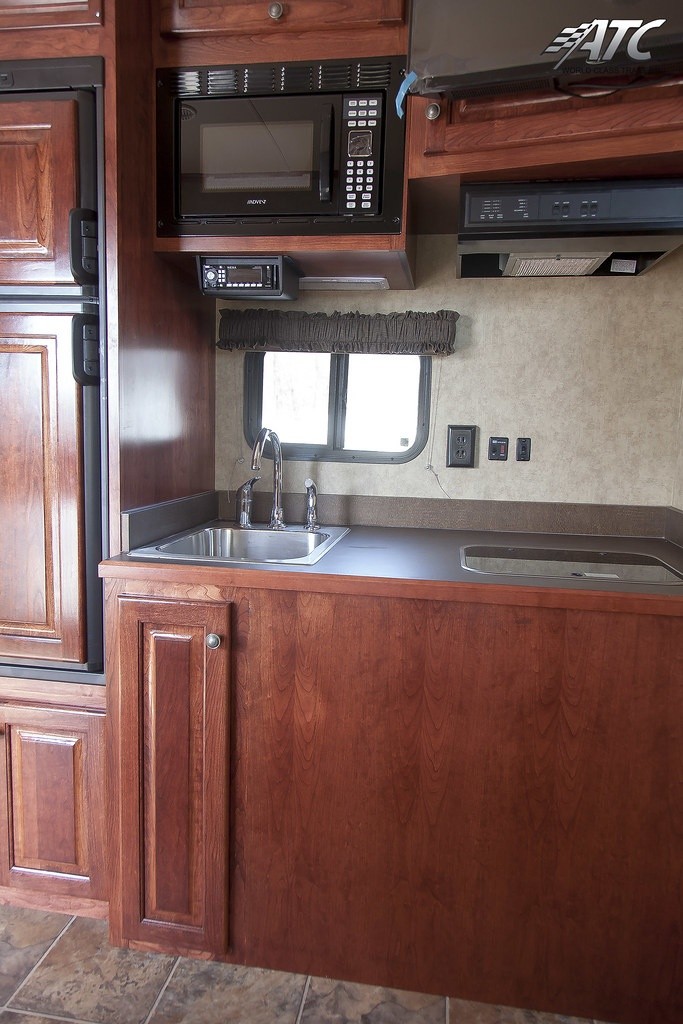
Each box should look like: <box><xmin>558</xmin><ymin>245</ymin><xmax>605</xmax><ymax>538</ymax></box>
<box><xmin>98</xmin><ymin>519</ymin><xmax>683</xmax><ymax>1023</ymax></box>
<box><xmin>0</xmin><ymin>0</ymin><xmax>215</xmax><ymax>921</ymax></box>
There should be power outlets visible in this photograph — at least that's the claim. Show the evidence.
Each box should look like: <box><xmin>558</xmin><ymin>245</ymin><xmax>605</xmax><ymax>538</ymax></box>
<box><xmin>446</xmin><ymin>425</ymin><xmax>477</xmax><ymax>468</ymax></box>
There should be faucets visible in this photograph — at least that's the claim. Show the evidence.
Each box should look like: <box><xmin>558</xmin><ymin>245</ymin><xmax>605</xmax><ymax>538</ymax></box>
<box><xmin>251</xmin><ymin>427</ymin><xmax>288</xmax><ymax>531</ymax></box>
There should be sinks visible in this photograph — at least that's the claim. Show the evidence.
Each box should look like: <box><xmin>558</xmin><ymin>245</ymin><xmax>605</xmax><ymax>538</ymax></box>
<box><xmin>126</xmin><ymin>518</ymin><xmax>352</xmax><ymax>567</ymax></box>
